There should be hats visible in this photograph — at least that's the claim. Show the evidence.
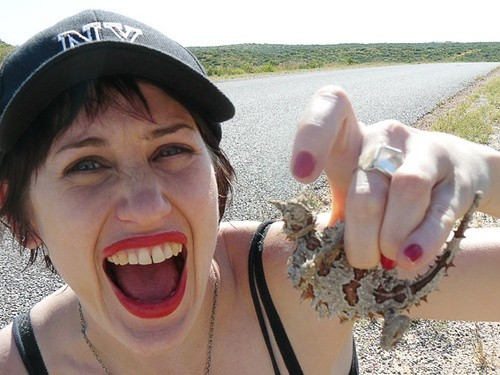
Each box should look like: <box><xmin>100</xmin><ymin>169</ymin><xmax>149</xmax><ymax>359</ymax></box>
<box><xmin>2</xmin><ymin>8</ymin><xmax>236</xmax><ymax>155</ymax></box>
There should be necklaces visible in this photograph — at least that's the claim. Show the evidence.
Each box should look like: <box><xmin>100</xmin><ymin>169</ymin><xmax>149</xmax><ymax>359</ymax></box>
<box><xmin>77</xmin><ymin>261</ymin><xmax>218</xmax><ymax>373</ymax></box>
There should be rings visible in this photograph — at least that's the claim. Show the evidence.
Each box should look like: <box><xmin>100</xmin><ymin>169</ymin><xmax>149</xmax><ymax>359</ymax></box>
<box><xmin>351</xmin><ymin>140</ymin><xmax>404</xmax><ymax>180</ymax></box>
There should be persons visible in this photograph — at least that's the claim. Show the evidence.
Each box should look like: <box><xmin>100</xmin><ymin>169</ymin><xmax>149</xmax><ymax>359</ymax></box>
<box><xmin>0</xmin><ymin>10</ymin><xmax>499</xmax><ymax>374</ymax></box>
